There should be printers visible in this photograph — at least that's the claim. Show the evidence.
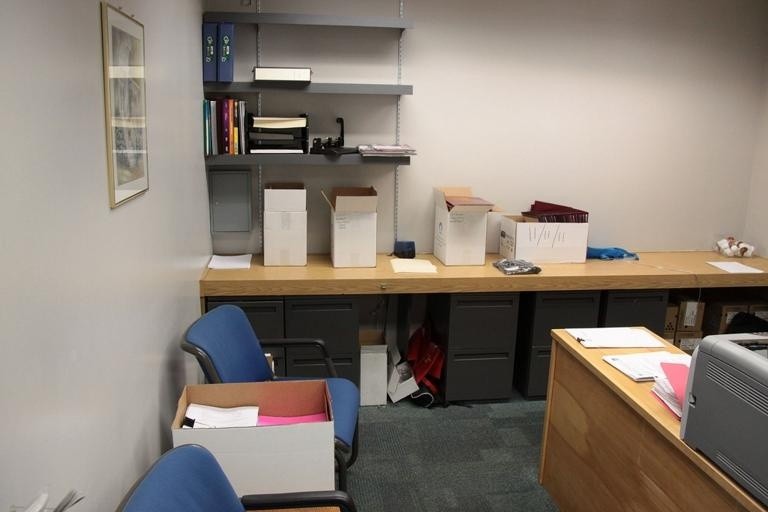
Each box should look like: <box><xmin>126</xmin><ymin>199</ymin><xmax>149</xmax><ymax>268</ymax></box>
<box><xmin>679</xmin><ymin>331</ymin><xmax>768</xmax><ymax>507</ymax></box>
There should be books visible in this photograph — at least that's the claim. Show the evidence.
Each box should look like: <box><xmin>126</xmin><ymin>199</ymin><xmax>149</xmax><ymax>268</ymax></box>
<box><xmin>602</xmin><ymin>349</ymin><xmax>676</xmax><ymax>383</ymax></box>
<box><xmin>202</xmin><ymin>22</ymin><xmax>313</xmax><ymax>156</ymax></box>
<box><xmin>356</xmin><ymin>143</ymin><xmax>418</xmax><ymax>159</ymax></box>
<box><xmin>371</xmin><ymin>142</ymin><xmax>417</xmax><ymax>156</ymax></box>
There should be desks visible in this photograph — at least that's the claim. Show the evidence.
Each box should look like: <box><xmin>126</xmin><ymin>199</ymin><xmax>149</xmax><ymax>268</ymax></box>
<box><xmin>538</xmin><ymin>325</ymin><xmax>766</xmax><ymax>512</ymax></box>
<box><xmin>199</xmin><ymin>251</ymin><xmax>768</xmax><ymax>407</ymax></box>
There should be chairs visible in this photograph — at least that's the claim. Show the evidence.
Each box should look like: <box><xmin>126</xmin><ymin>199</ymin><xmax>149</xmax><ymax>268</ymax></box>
<box><xmin>115</xmin><ymin>445</ymin><xmax>357</xmax><ymax>512</ymax></box>
<box><xmin>180</xmin><ymin>306</ymin><xmax>361</xmax><ymax>491</ymax></box>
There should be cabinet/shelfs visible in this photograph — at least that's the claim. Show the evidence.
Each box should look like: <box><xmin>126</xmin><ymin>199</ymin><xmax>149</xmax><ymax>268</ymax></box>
<box><xmin>203</xmin><ymin>11</ymin><xmax>413</xmax><ymax>166</ymax></box>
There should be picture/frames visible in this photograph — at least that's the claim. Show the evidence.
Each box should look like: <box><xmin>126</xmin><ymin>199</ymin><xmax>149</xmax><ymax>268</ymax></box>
<box><xmin>102</xmin><ymin>1</ymin><xmax>149</xmax><ymax>208</ymax></box>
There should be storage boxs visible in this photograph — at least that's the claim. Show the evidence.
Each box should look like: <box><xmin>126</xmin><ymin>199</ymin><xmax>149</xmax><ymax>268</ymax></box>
<box><xmin>500</xmin><ymin>213</ymin><xmax>589</xmax><ymax>264</ymax></box>
<box><xmin>665</xmin><ymin>297</ymin><xmax>767</xmax><ymax>356</ymax></box>
<box><xmin>264</xmin><ymin>182</ymin><xmax>308</xmax><ymax>267</ymax></box>
<box><xmin>434</xmin><ymin>185</ymin><xmax>504</xmax><ymax>266</ymax></box>
<box><xmin>320</xmin><ymin>185</ymin><xmax>378</xmax><ymax>268</ymax></box>
<box><xmin>169</xmin><ymin>379</ymin><xmax>336</xmax><ymax>499</ymax></box>
<box><xmin>358</xmin><ymin>325</ymin><xmax>387</xmax><ymax>406</ymax></box>
